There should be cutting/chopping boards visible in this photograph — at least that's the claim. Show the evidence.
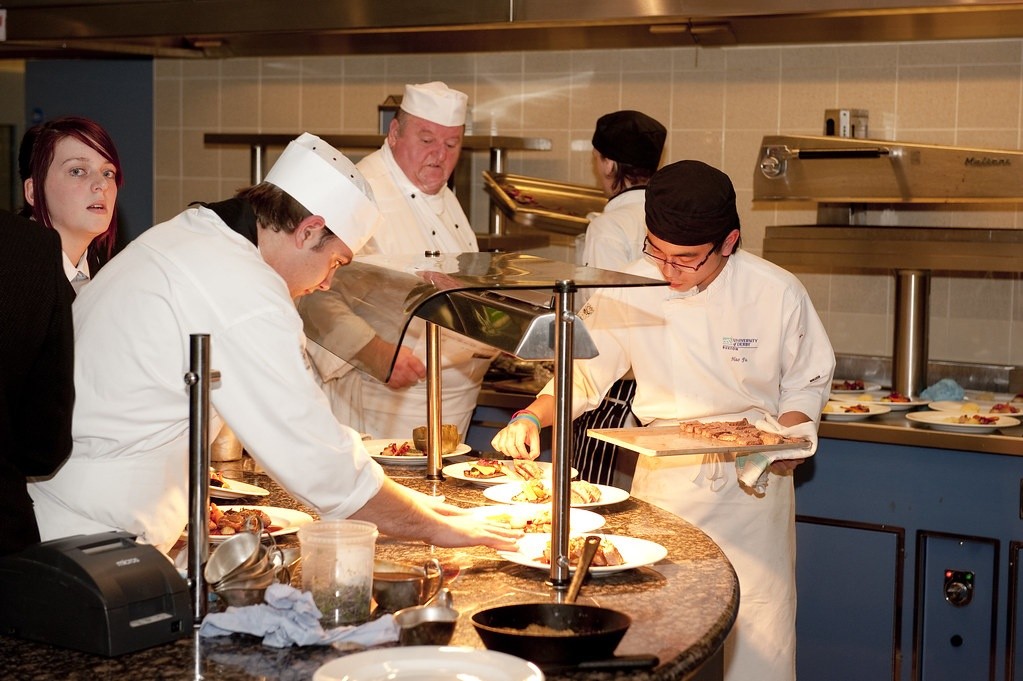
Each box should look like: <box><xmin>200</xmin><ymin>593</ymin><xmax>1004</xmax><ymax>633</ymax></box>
<box><xmin>585</xmin><ymin>425</ymin><xmax>813</xmax><ymax>458</ymax></box>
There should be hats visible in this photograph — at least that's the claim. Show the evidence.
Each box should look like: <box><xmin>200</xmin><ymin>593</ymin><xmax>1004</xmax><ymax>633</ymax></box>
<box><xmin>644</xmin><ymin>160</ymin><xmax>740</xmax><ymax>246</ymax></box>
<box><xmin>592</xmin><ymin>109</ymin><xmax>668</xmax><ymax>168</ymax></box>
<box><xmin>400</xmin><ymin>81</ymin><xmax>469</xmax><ymax>127</ymax></box>
<box><xmin>264</xmin><ymin>131</ymin><xmax>382</xmax><ymax>256</ymax></box>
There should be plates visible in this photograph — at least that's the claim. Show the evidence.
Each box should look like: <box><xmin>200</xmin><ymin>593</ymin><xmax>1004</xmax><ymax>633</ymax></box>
<box><xmin>823</xmin><ymin>379</ymin><xmax>1023</xmax><ymax>435</ymax></box>
<box><xmin>496</xmin><ymin>533</ymin><xmax>668</xmax><ymax>576</ymax></box>
<box><xmin>483</xmin><ymin>482</ymin><xmax>633</xmax><ymax>512</ymax></box>
<box><xmin>442</xmin><ymin>459</ymin><xmax>579</xmax><ymax>486</ymax></box>
<box><xmin>363</xmin><ymin>438</ymin><xmax>472</xmax><ymax>465</ymax></box>
<box><xmin>466</xmin><ymin>503</ymin><xmax>608</xmax><ymax>533</ymax></box>
<box><xmin>179</xmin><ymin>504</ymin><xmax>315</xmax><ymax>544</ymax></box>
<box><xmin>209</xmin><ymin>478</ymin><xmax>270</xmax><ymax>500</ymax></box>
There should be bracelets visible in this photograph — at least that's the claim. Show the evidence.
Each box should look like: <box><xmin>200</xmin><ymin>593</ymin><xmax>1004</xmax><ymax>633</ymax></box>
<box><xmin>508</xmin><ymin>410</ymin><xmax>541</xmax><ymax>434</ymax></box>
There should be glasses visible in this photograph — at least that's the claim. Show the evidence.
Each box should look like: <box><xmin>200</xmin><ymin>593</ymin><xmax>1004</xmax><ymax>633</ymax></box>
<box><xmin>642</xmin><ymin>236</ymin><xmax>724</xmax><ymax>274</ymax></box>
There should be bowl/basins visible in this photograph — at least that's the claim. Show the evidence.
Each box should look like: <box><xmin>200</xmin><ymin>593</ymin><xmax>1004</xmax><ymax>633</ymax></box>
<box><xmin>469</xmin><ymin>602</ymin><xmax>634</xmax><ymax>678</ymax></box>
<box><xmin>311</xmin><ymin>644</ymin><xmax>545</xmax><ymax>681</ymax></box>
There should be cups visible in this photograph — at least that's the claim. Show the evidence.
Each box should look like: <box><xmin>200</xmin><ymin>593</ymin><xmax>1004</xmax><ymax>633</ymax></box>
<box><xmin>201</xmin><ymin>514</ymin><xmax>291</xmax><ymax>608</ymax></box>
<box><xmin>372</xmin><ymin>557</ymin><xmax>460</xmax><ymax>647</ymax></box>
<box><xmin>296</xmin><ymin>518</ymin><xmax>380</xmax><ymax>628</ymax></box>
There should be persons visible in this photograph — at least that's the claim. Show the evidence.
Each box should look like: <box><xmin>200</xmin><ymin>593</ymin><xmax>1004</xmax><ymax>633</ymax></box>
<box><xmin>572</xmin><ymin>110</ymin><xmax>667</xmax><ymax>493</ymax></box>
<box><xmin>290</xmin><ymin>81</ymin><xmax>500</xmax><ymax>445</ymax></box>
<box><xmin>492</xmin><ymin>160</ymin><xmax>836</xmax><ymax>681</ymax></box>
<box><xmin>18</xmin><ymin>116</ymin><xmax>123</xmax><ymax>294</ymax></box>
<box><xmin>0</xmin><ymin>205</ymin><xmax>76</xmax><ymax>547</ymax></box>
<box><xmin>28</xmin><ymin>132</ymin><xmax>524</xmax><ymax>554</ymax></box>
